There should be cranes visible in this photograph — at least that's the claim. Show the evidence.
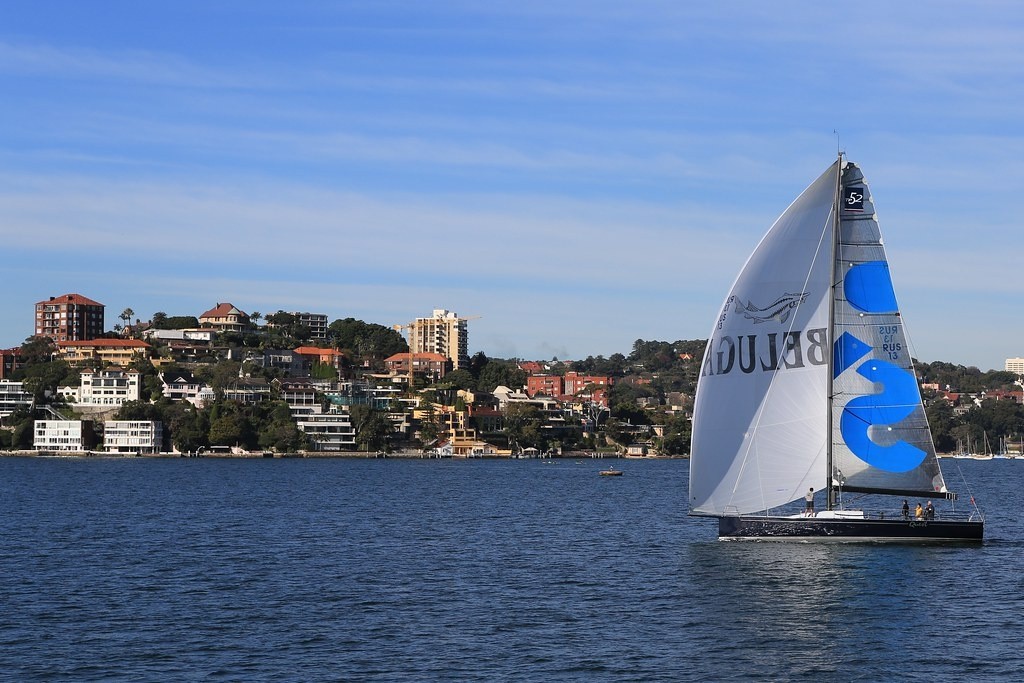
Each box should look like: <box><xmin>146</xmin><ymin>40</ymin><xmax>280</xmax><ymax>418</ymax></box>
<box><xmin>393</xmin><ymin>315</ymin><xmax>483</xmax><ymax>399</ymax></box>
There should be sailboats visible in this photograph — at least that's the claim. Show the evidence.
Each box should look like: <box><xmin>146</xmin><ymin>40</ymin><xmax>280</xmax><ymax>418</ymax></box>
<box><xmin>687</xmin><ymin>129</ymin><xmax>985</xmax><ymax>542</ymax></box>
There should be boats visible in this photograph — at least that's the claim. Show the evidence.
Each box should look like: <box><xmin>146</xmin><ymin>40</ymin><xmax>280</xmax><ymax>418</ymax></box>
<box><xmin>600</xmin><ymin>470</ymin><xmax>623</xmax><ymax>476</ymax></box>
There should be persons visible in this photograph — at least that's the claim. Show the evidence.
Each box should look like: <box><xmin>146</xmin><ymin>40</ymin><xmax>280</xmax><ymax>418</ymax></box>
<box><xmin>901</xmin><ymin>500</ymin><xmax>909</xmax><ymax>517</ymax></box>
<box><xmin>804</xmin><ymin>487</ymin><xmax>815</xmax><ymax>517</ymax></box>
<box><xmin>914</xmin><ymin>503</ymin><xmax>924</xmax><ymax>517</ymax></box>
<box><xmin>924</xmin><ymin>500</ymin><xmax>935</xmax><ymax>520</ymax></box>
